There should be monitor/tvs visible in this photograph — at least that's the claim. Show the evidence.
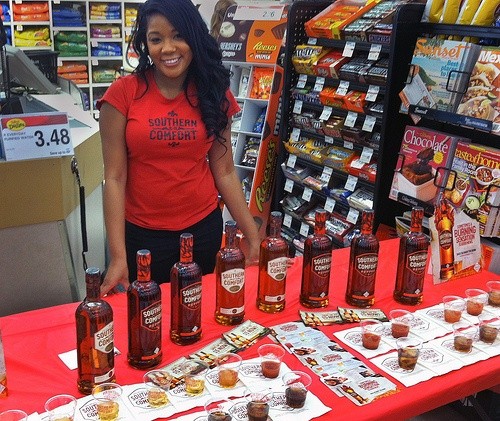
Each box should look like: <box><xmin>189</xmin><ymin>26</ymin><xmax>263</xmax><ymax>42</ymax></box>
<box><xmin>3</xmin><ymin>44</ymin><xmax>54</xmax><ymax>94</ymax></box>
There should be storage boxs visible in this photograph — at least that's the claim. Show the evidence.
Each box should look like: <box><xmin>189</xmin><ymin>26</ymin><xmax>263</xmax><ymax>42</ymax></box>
<box><xmin>341</xmin><ymin>0</ymin><xmax>408</xmax><ymax>42</ymax></box>
<box><xmin>304</xmin><ymin>0</ymin><xmax>381</xmax><ymax>40</ymax></box>
<box><xmin>366</xmin><ymin>10</ymin><xmax>397</xmax><ymax>42</ymax></box>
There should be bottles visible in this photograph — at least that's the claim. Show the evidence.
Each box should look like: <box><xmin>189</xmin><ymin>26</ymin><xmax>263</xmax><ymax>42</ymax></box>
<box><xmin>345</xmin><ymin>208</ymin><xmax>380</xmax><ymax>308</ymax></box>
<box><xmin>436</xmin><ymin>199</ymin><xmax>456</xmax><ymax>279</ymax></box>
<box><xmin>255</xmin><ymin>210</ymin><xmax>289</xmax><ymax>315</ymax></box>
<box><xmin>127</xmin><ymin>249</ymin><xmax>163</xmax><ymax>370</ymax></box>
<box><xmin>154</xmin><ymin>307</ymin><xmax>367</xmax><ymax>419</ymax></box>
<box><xmin>299</xmin><ymin>208</ymin><xmax>332</xmax><ymax>309</ymax></box>
<box><xmin>169</xmin><ymin>232</ymin><xmax>203</xmax><ymax>346</ymax></box>
<box><xmin>393</xmin><ymin>205</ymin><xmax>430</xmax><ymax>306</ymax></box>
<box><xmin>75</xmin><ymin>266</ymin><xmax>117</xmax><ymax>395</ymax></box>
<box><xmin>214</xmin><ymin>220</ymin><xmax>246</xmax><ymax>327</ymax></box>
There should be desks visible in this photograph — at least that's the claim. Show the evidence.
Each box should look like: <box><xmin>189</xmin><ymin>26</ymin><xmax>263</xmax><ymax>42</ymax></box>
<box><xmin>1</xmin><ymin>238</ymin><xmax>500</xmax><ymax>421</ymax></box>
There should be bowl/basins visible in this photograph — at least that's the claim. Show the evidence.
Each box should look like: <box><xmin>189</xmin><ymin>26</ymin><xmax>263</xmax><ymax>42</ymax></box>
<box><xmin>455</xmin><ymin>166</ymin><xmax>494</xmax><ymax>194</ymax></box>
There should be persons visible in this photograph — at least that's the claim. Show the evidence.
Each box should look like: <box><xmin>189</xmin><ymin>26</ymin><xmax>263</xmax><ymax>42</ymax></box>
<box><xmin>94</xmin><ymin>0</ymin><xmax>295</xmax><ymax>299</ymax></box>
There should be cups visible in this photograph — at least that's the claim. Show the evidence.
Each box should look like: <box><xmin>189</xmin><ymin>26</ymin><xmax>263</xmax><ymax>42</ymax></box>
<box><xmin>282</xmin><ymin>370</ymin><xmax>313</xmax><ymax>409</ymax></box>
<box><xmin>44</xmin><ymin>395</ymin><xmax>77</xmax><ymax>421</ymax></box>
<box><xmin>257</xmin><ymin>343</ymin><xmax>285</xmax><ymax>379</ymax></box>
<box><xmin>216</xmin><ymin>352</ymin><xmax>243</xmax><ymax>388</ymax></box>
<box><xmin>0</xmin><ymin>409</ymin><xmax>28</xmax><ymax>421</ymax></box>
<box><xmin>180</xmin><ymin>359</ymin><xmax>209</xmax><ymax>396</ymax></box>
<box><xmin>398</xmin><ymin>165</ymin><xmax>441</xmax><ymax>203</ymax></box>
<box><xmin>92</xmin><ymin>382</ymin><xmax>123</xmax><ymax>421</ymax></box>
<box><xmin>360</xmin><ymin>319</ymin><xmax>385</xmax><ymax>351</ymax></box>
<box><xmin>204</xmin><ymin>397</ymin><xmax>236</xmax><ymax>421</ymax></box>
<box><xmin>442</xmin><ymin>280</ymin><xmax>500</xmax><ymax>355</ymax></box>
<box><xmin>243</xmin><ymin>386</ymin><xmax>274</xmax><ymax>421</ymax></box>
<box><xmin>388</xmin><ymin>310</ymin><xmax>414</xmax><ymax>340</ymax></box>
<box><xmin>396</xmin><ymin>336</ymin><xmax>424</xmax><ymax>373</ymax></box>
<box><xmin>143</xmin><ymin>369</ymin><xmax>171</xmax><ymax>408</ymax></box>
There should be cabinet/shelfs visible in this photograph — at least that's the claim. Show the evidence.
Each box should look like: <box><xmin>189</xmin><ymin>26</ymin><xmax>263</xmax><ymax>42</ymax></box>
<box><xmin>0</xmin><ymin>0</ymin><xmax>500</xmax><ymax>275</ymax></box>
